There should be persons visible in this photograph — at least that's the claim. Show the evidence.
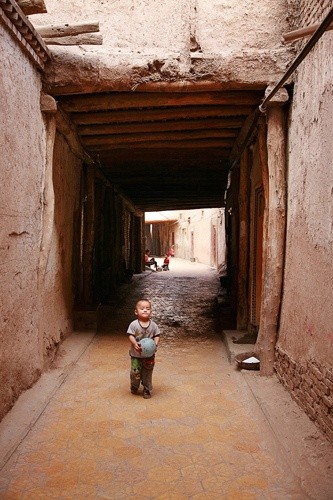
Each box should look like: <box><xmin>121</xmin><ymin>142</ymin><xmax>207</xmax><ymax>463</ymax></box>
<box><xmin>162</xmin><ymin>254</ymin><xmax>170</xmax><ymax>271</ymax></box>
<box><xmin>145</xmin><ymin>249</ymin><xmax>158</xmax><ymax>271</ymax></box>
<box><xmin>128</xmin><ymin>299</ymin><xmax>161</xmax><ymax>398</ymax></box>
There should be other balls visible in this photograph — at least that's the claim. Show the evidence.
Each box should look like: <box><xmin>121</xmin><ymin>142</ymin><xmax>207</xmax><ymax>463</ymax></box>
<box><xmin>139</xmin><ymin>338</ymin><xmax>156</xmax><ymax>357</ymax></box>
